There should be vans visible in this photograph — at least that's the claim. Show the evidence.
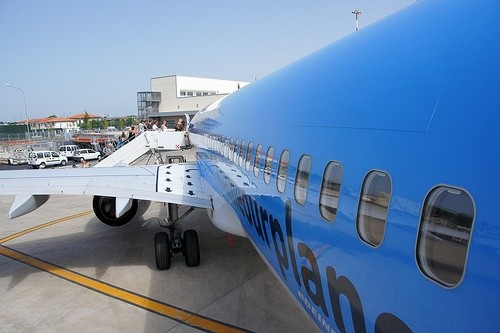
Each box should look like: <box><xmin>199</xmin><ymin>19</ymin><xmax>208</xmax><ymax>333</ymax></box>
<box><xmin>27</xmin><ymin>150</ymin><xmax>68</xmax><ymax>169</ymax></box>
<box><xmin>58</xmin><ymin>145</ymin><xmax>80</xmax><ymax>158</ymax></box>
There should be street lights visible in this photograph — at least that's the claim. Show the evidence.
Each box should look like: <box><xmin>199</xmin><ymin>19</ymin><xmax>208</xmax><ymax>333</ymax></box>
<box><xmin>2</xmin><ymin>82</ymin><xmax>30</xmax><ymax>140</ymax></box>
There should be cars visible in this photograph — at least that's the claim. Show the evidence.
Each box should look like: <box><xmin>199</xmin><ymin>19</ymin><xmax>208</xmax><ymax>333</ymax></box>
<box><xmin>74</xmin><ymin>149</ymin><xmax>102</xmax><ymax>162</ymax></box>
<box><xmin>14</xmin><ymin>148</ymin><xmax>26</xmax><ymax>153</ymax></box>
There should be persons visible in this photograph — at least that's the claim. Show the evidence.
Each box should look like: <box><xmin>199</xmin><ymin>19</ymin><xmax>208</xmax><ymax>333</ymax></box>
<box><xmin>72</xmin><ymin>119</ymin><xmax>186</xmax><ymax>168</ymax></box>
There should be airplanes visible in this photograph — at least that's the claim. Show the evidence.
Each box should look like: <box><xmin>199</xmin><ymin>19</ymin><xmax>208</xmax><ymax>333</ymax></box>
<box><xmin>0</xmin><ymin>0</ymin><xmax>499</xmax><ymax>333</ymax></box>
